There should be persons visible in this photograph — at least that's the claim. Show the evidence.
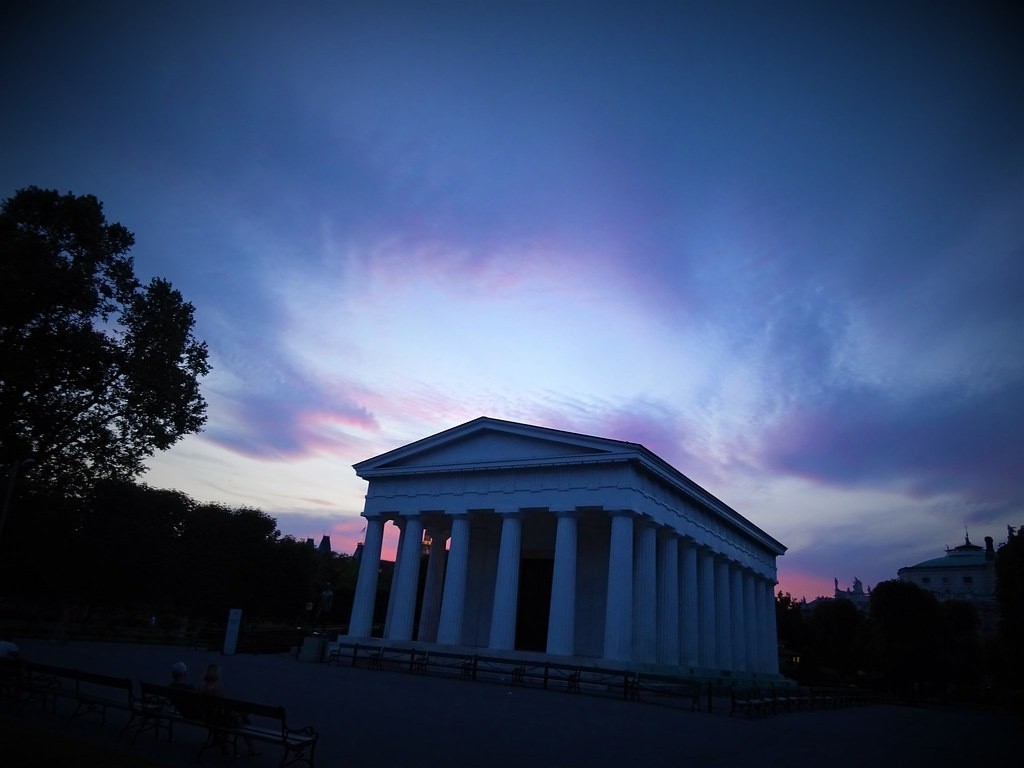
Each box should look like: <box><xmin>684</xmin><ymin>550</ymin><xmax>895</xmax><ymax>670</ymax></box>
<box><xmin>0</xmin><ymin>629</ymin><xmax>19</xmax><ymax>661</ymax></box>
<box><xmin>200</xmin><ymin>665</ymin><xmax>263</xmax><ymax>756</ymax></box>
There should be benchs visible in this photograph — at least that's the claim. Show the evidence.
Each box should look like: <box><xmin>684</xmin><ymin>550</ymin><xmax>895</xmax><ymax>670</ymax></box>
<box><xmin>66</xmin><ymin>670</ymin><xmax>166</xmax><ymax>748</ymax></box>
<box><xmin>329</xmin><ymin>643</ymin><xmax>876</xmax><ymax>721</ymax></box>
<box><xmin>167</xmin><ymin>686</ymin><xmax>319</xmax><ymax>768</ymax></box>
<box><xmin>0</xmin><ymin>658</ymin><xmax>71</xmax><ymax>714</ymax></box>
